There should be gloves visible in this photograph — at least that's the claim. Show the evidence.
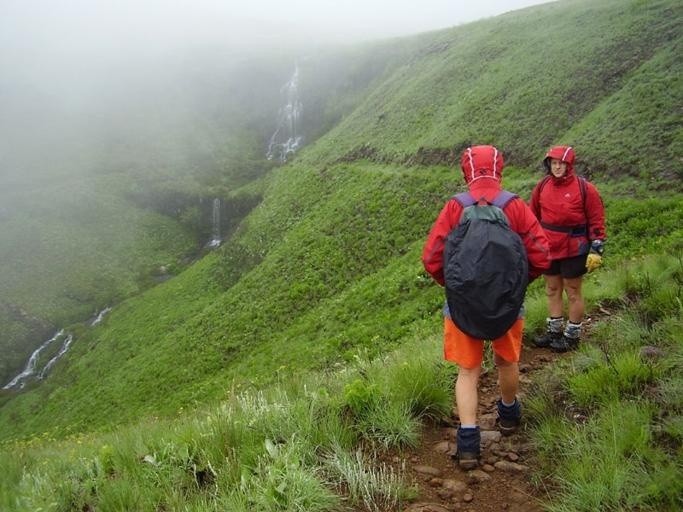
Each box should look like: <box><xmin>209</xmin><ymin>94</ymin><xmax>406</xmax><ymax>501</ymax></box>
<box><xmin>585</xmin><ymin>253</ymin><xmax>604</xmax><ymax>275</ymax></box>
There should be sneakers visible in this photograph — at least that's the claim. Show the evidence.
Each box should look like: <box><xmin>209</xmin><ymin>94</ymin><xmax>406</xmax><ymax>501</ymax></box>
<box><xmin>531</xmin><ymin>331</ymin><xmax>581</xmax><ymax>353</ymax></box>
<box><xmin>496</xmin><ymin>395</ymin><xmax>521</xmax><ymax>436</ymax></box>
<box><xmin>455</xmin><ymin>423</ymin><xmax>482</xmax><ymax>467</ymax></box>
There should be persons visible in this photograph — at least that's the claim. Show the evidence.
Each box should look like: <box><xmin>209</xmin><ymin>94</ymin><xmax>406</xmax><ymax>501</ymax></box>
<box><xmin>422</xmin><ymin>144</ymin><xmax>552</xmax><ymax>471</ymax></box>
<box><xmin>529</xmin><ymin>145</ymin><xmax>605</xmax><ymax>352</ymax></box>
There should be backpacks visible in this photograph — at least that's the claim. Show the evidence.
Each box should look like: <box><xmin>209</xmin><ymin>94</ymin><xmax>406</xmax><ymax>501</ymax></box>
<box><xmin>442</xmin><ymin>190</ymin><xmax>530</xmax><ymax>341</ymax></box>
